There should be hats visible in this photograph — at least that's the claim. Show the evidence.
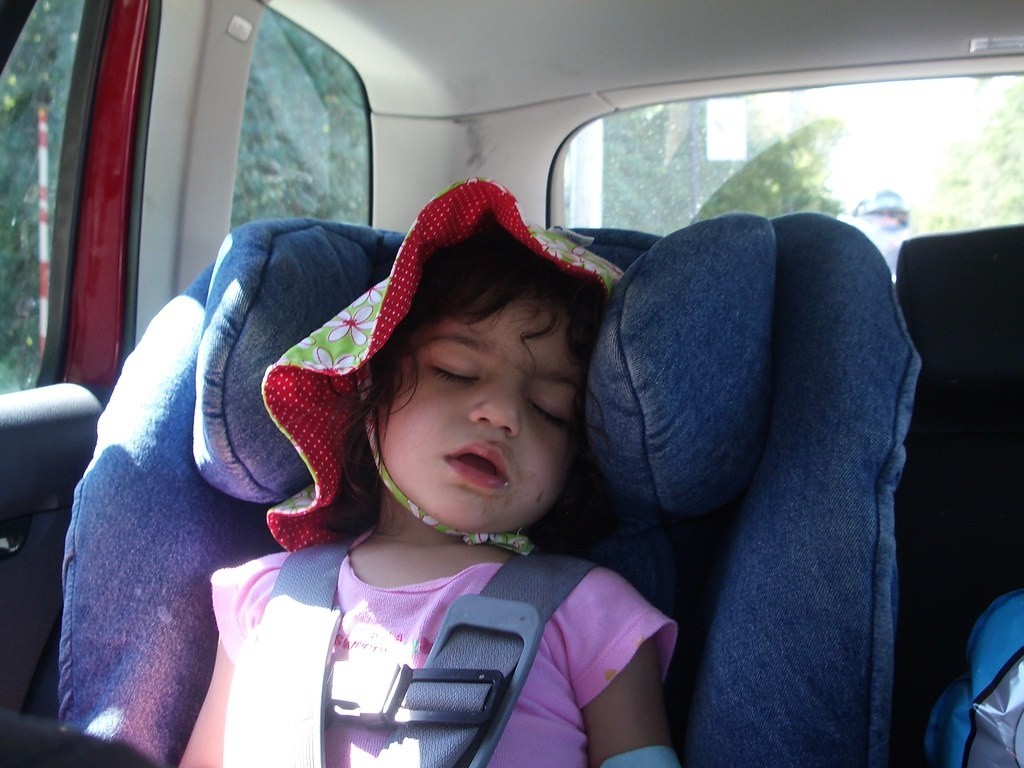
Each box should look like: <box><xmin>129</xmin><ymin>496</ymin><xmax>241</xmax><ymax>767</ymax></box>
<box><xmin>260</xmin><ymin>175</ymin><xmax>629</xmax><ymax>555</ymax></box>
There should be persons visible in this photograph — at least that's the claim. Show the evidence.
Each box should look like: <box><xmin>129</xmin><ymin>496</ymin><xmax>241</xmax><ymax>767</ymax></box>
<box><xmin>173</xmin><ymin>173</ymin><xmax>693</xmax><ymax>768</ymax></box>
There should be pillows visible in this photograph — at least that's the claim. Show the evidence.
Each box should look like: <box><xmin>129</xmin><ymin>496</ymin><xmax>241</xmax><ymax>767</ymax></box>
<box><xmin>193</xmin><ymin>212</ymin><xmax>776</xmax><ymax>513</ymax></box>
